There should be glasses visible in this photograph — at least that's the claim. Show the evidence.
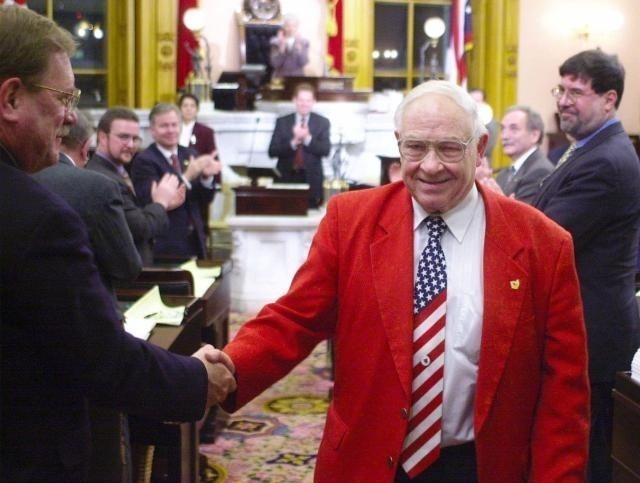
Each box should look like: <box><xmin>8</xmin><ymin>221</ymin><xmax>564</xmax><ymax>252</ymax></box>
<box><xmin>396</xmin><ymin>131</ymin><xmax>476</xmax><ymax>163</ymax></box>
<box><xmin>31</xmin><ymin>79</ymin><xmax>83</xmax><ymax>114</ymax></box>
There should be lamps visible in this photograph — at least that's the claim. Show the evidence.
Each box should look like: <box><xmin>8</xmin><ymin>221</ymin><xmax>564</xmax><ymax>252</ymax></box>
<box><xmin>423</xmin><ymin>18</ymin><xmax>447</xmax><ymax>80</ymax></box>
<box><xmin>182</xmin><ymin>8</ymin><xmax>212</xmax><ymax>100</ymax></box>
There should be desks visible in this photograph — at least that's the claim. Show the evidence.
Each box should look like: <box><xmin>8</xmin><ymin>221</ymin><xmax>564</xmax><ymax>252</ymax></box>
<box><xmin>121</xmin><ymin>257</ymin><xmax>231</xmax><ymax>483</ymax></box>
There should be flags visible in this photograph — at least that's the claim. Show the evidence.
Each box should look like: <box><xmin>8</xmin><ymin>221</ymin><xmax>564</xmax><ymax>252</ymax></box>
<box><xmin>451</xmin><ymin>0</ymin><xmax>474</xmax><ymax>86</ymax></box>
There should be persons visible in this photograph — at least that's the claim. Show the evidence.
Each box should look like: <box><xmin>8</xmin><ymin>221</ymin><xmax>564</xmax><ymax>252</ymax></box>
<box><xmin>29</xmin><ymin>95</ymin><xmax>221</xmax><ymax>300</ymax></box>
<box><xmin>268</xmin><ymin>17</ymin><xmax>309</xmax><ymax>78</ymax></box>
<box><xmin>0</xmin><ymin>4</ymin><xmax>238</xmax><ymax>483</ymax></box>
<box><xmin>529</xmin><ymin>49</ymin><xmax>640</xmax><ymax>482</ymax></box>
<box><xmin>467</xmin><ymin>89</ymin><xmax>498</xmax><ymax>156</ymax></box>
<box><xmin>268</xmin><ymin>82</ymin><xmax>330</xmax><ymax>209</ymax></box>
<box><xmin>205</xmin><ymin>77</ymin><xmax>591</xmax><ymax>483</ymax></box>
<box><xmin>494</xmin><ymin>103</ymin><xmax>556</xmax><ymax>206</ymax></box>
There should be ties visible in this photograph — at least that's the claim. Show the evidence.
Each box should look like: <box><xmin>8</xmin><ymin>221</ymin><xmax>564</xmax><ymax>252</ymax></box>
<box><xmin>539</xmin><ymin>143</ymin><xmax>578</xmax><ymax>188</ymax></box>
<box><xmin>172</xmin><ymin>154</ymin><xmax>182</xmax><ymax>175</ymax></box>
<box><xmin>397</xmin><ymin>214</ymin><xmax>449</xmax><ymax>479</ymax></box>
<box><xmin>293</xmin><ymin>118</ymin><xmax>307</xmax><ymax>169</ymax></box>
